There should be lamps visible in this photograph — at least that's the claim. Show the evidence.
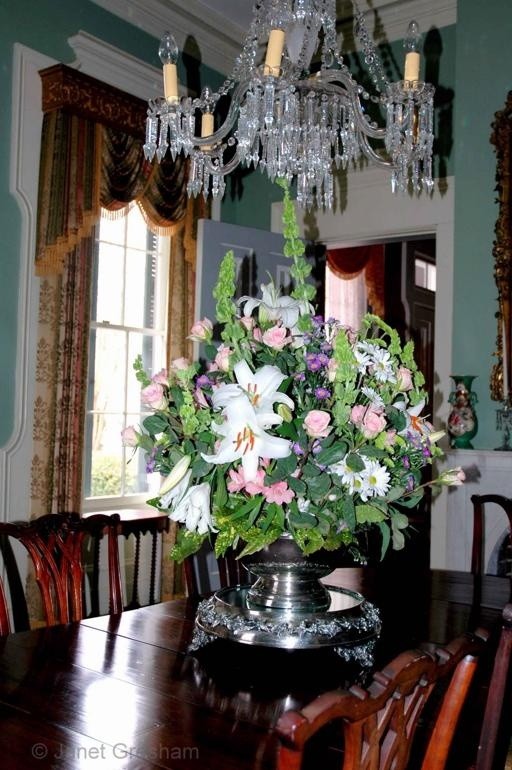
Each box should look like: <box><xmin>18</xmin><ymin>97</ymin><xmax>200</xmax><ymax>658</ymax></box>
<box><xmin>143</xmin><ymin>0</ymin><xmax>448</xmax><ymax>218</ymax></box>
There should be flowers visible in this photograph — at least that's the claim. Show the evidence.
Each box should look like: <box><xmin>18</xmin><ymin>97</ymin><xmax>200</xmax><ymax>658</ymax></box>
<box><xmin>119</xmin><ymin>181</ymin><xmax>470</xmax><ymax>573</ymax></box>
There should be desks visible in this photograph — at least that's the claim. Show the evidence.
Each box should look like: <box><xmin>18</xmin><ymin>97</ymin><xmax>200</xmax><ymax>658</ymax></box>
<box><xmin>1</xmin><ymin>562</ymin><xmax>512</xmax><ymax>769</ymax></box>
<box><xmin>82</xmin><ymin>506</ymin><xmax>170</xmax><ymax>613</ymax></box>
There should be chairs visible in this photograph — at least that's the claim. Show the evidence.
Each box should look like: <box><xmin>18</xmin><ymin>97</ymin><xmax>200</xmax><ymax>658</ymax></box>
<box><xmin>470</xmin><ymin>492</ymin><xmax>511</xmax><ymax>578</ymax></box>
<box><xmin>264</xmin><ymin>641</ymin><xmax>475</xmax><ymax>770</ymax></box>
<box><xmin>427</xmin><ymin>601</ymin><xmax>511</xmax><ymax>770</ymax></box>
<box><xmin>0</xmin><ymin>511</ymin><xmax>126</xmax><ymax>633</ymax></box>
